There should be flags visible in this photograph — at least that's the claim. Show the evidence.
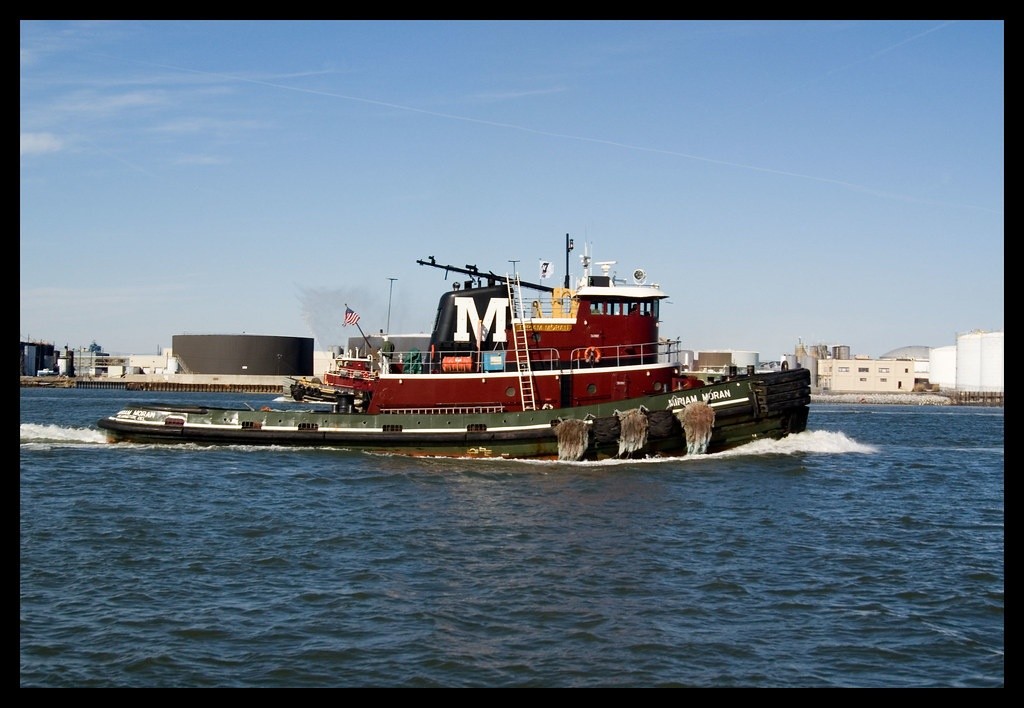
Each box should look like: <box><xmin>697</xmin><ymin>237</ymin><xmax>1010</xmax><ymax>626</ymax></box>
<box><xmin>342</xmin><ymin>308</ymin><xmax>360</xmax><ymax>327</ymax></box>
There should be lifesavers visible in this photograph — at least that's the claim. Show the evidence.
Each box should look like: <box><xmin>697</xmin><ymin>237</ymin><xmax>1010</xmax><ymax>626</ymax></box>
<box><xmin>306</xmin><ymin>387</ymin><xmax>320</xmax><ymax>395</ymax></box>
<box><xmin>533</xmin><ymin>301</ymin><xmax>539</xmax><ymax>307</ymax></box>
<box><xmin>297</xmin><ymin>384</ymin><xmax>305</xmax><ymax>391</ymax></box>
<box><xmin>584</xmin><ymin>346</ymin><xmax>602</xmax><ymax>363</ymax></box>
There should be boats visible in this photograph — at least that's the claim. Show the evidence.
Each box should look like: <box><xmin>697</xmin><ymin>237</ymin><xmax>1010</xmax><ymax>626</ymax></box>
<box><xmin>96</xmin><ymin>232</ymin><xmax>813</xmax><ymax>464</ymax></box>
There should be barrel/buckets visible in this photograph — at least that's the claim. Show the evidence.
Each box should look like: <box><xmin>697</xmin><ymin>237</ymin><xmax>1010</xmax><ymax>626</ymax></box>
<box><xmin>747</xmin><ymin>365</ymin><xmax>754</xmax><ymax>375</ymax></box>
<box><xmin>729</xmin><ymin>366</ymin><xmax>737</xmax><ymax>376</ymax></box>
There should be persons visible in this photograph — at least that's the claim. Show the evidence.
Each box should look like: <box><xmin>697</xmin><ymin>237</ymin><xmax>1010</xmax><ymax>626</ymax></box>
<box><xmin>431</xmin><ymin>338</ymin><xmax>440</xmax><ymax>373</ymax></box>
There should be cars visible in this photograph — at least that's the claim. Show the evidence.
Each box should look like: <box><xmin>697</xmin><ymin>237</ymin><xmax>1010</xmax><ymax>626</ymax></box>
<box><xmin>37</xmin><ymin>368</ymin><xmax>58</xmax><ymax>378</ymax></box>
